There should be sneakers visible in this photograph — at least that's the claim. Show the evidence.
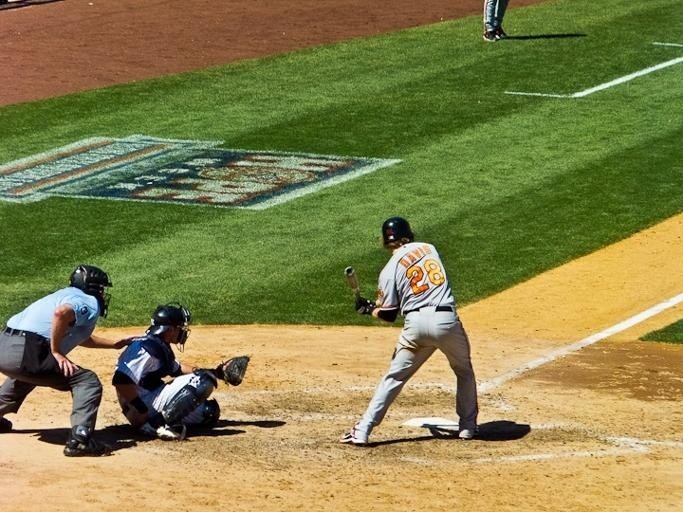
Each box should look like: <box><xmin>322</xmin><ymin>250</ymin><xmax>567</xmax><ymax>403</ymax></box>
<box><xmin>64</xmin><ymin>434</ymin><xmax>113</xmax><ymax>458</ymax></box>
<box><xmin>481</xmin><ymin>26</ymin><xmax>510</xmax><ymax>43</ymax></box>
<box><xmin>137</xmin><ymin>422</ymin><xmax>181</xmax><ymax>442</ymax></box>
<box><xmin>337</xmin><ymin>431</ymin><xmax>369</xmax><ymax>445</ymax></box>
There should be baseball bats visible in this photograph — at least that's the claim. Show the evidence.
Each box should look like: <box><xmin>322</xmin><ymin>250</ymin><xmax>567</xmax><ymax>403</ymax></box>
<box><xmin>344</xmin><ymin>266</ymin><xmax>362</xmax><ymax>300</ymax></box>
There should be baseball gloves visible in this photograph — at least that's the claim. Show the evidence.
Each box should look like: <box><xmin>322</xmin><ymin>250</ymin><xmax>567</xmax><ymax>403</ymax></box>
<box><xmin>214</xmin><ymin>355</ymin><xmax>249</xmax><ymax>385</ymax></box>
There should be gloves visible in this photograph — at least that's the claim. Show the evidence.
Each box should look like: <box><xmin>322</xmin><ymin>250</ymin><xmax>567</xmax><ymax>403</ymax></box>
<box><xmin>351</xmin><ymin>294</ymin><xmax>377</xmax><ymax>316</ymax></box>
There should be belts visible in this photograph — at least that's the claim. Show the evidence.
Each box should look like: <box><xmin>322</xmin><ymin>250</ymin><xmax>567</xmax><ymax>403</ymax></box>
<box><xmin>2</xmin><ymin>325</ymin><xmax>42</xmax><ymax>343</ymax></box>
<box><xmin>405</xmin><ymin>306</ymin><xmax>453</xmax><ymax>315</ymax></box>
<box><xmin>122</xmin><ymin>405</ymin><xmax>130</xmax><ymax>417</ymax></box>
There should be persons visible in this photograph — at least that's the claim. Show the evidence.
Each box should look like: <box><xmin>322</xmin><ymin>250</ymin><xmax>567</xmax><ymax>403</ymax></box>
<box><xmin>482</xmin><ymin>0</ymin><xmax>512</xmax><ymax>43</ymax></box>
<box><xmin>110</xmin><ymin>301</ymin><xmax>251</xmax><ymax>442</ymax></box>
<box><xmin>335</xmin><ymin>215</ymin><xmax>482</xmax><ymax>446</ymax></box>
<box><xmin>0</xmin><ymin>263</ymin><xmax>141</xmax><ymax>458</ymax></box>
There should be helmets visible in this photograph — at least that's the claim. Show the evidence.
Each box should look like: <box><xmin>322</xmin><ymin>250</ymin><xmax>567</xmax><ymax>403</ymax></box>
<box><xmin>144</xmin><ymin>304</ymin><xmax>191</xmax><ymax>339</ymax></box>
<box><xmin>381</xmin><ymin>217</ymin><xmax>414</xmax><ymax>248</ymax></box>
<box><xmin>70</xmin><ymin>264</ymin><xmax>110</xmax><ymax>295</ymax></box>
